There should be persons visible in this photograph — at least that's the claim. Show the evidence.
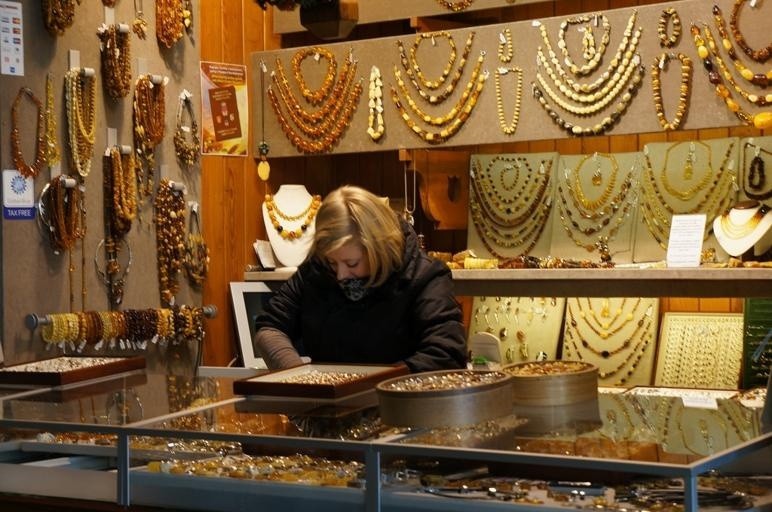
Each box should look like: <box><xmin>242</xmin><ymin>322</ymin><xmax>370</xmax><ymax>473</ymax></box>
<box><xmin>253</xmin><ymin>182</ymin><xmax>470</xmax><ymax>374</ymax></box>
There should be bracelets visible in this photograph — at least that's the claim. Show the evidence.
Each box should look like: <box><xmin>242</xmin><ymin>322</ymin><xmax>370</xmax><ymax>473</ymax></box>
<box><xmin>40</xmin><ymin>303</ymin><xmax>206</xmax><ymax>351</ymax></box>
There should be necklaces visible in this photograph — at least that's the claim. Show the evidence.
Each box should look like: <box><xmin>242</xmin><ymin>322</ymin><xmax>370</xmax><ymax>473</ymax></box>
<box><xmin>562</xmin><ymin>296</ymin><xmax>654</xmax><ymax>390</ymax></box>
<box><xmin>255</xmin><ymin>0</ymin><xmax>771</xmax><ymax>261</ymax></box>
<box><xmin>9</xmin><ymin>0</ymin><xmax>211</xmax><ymax>309</ymax></box>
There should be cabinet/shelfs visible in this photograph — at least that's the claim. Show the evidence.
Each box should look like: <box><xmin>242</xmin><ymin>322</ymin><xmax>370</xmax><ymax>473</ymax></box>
<box><xmin>1</xmin><ymin>362</ymin><xmax>771</xmax><ymax>510</ymax></box>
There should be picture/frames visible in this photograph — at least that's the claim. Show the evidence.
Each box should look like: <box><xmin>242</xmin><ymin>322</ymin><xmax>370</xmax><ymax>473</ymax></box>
<box><xmin>230</xmin><ymin>280</ymin><xmax>313</xmax><ymax>370</ymax></box>
